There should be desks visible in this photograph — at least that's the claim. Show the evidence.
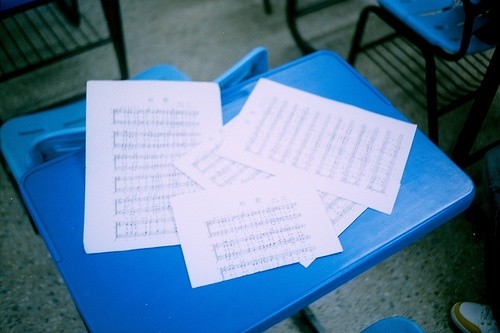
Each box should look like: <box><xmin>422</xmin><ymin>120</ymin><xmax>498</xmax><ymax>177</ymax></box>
<box><xmin>18</xmin><ymin>48</ymin><xmax>476</xmax><ymax>333</ymax></box>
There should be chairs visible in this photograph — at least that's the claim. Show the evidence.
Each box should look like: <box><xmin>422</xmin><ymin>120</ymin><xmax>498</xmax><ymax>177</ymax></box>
<box><xmin>0</xmin><ymin>46</ymin><xmax>273</xmax><ymax>233</ymax></box>
<box><xmin>348</xmin><ymin>1</ymin><xmax>500</xmax><ymax>175</ymax></box>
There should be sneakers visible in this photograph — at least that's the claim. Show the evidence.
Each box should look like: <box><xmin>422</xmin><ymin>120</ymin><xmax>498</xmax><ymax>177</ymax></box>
<box><xmin>450</xmin><ymin>301</ymin><xmax>500</xmax><ymax>333</ymax></box>
